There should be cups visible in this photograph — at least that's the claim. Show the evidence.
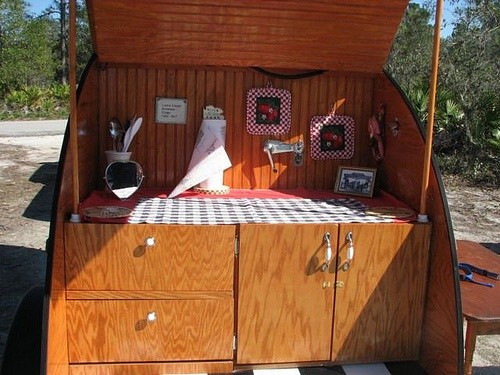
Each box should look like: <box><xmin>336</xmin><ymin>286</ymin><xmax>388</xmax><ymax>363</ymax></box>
<box><xmin>105</xmin><ymin>150</ymin><xmax>133</xmax><ymax>167</ymax></box>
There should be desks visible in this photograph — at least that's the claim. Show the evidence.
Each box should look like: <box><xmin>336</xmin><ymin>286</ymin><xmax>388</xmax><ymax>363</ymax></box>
<box><xmin>456</xmin><ymin>240</ymin><xmax>500</xmax><ymax>375</ymax></box>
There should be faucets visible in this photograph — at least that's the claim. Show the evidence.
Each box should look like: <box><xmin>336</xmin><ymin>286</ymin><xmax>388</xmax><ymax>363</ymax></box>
<box><xmin>262</xmin><ymin>139</ymin><xmax>303</xmax><ymax>174</ymax></box>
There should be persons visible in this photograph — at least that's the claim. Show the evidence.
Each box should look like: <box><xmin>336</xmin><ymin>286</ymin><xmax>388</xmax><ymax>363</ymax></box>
<box><xmin>344</xmin><ymin>175</ymin><xmax>372</xmax><ymax>192</ymax></box>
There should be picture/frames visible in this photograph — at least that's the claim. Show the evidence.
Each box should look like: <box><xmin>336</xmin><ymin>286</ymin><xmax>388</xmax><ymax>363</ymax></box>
<box><xmin>333</xmin><ymin>165</ymin><xmax>377</xmax><ymax>198</ymax></box>
<box><xmin>246</xmin><ymin>88</ymin><xmax>291</xmax><ymax>134</ymax></box>
<box><xmin>310</xmin><ymin>115</ymin><xmax>354</xmax><ymax>160</ymax></box>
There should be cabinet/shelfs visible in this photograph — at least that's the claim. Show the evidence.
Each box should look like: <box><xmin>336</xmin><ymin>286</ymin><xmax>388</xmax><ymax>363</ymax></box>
<box><xmin>63</xmin><ymin>222</ymin><xmax>235</xmax><ymax>374</ymax></box>
<box><xmin>236</xmin><ymin>222</ymin><xmax>431</xmax><ymax>369</ymax></box>
